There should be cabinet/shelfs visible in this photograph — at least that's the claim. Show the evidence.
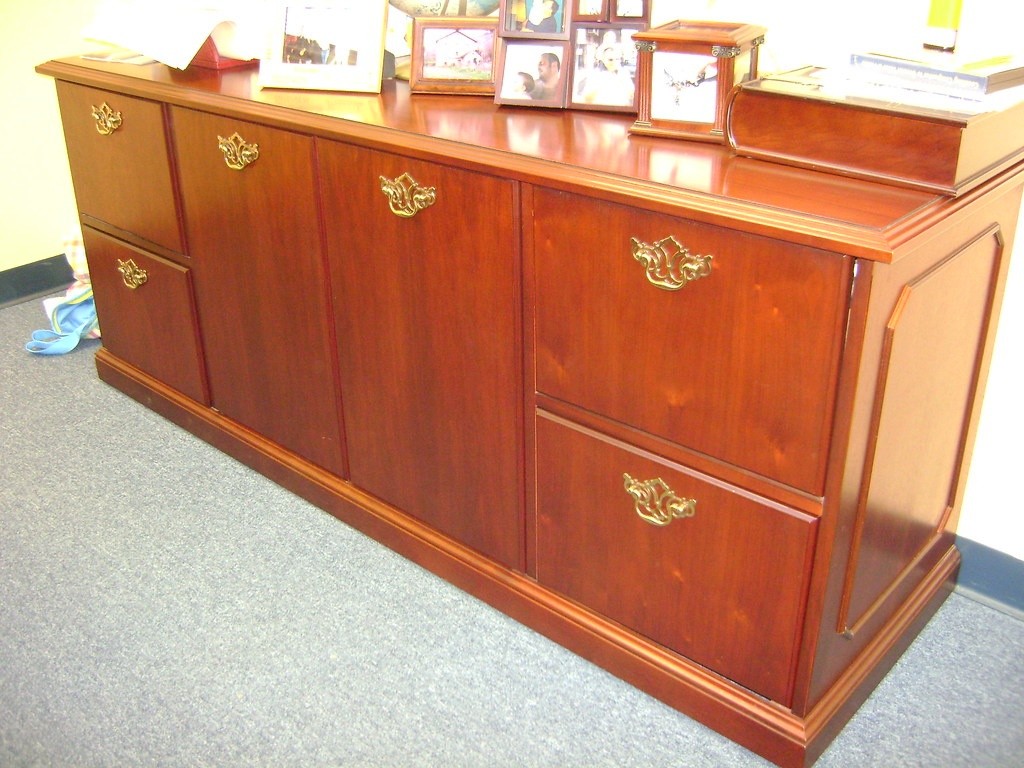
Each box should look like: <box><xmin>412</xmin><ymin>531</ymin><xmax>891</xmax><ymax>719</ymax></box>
<box><xmin>524</xmin><ymin>153</ymin><xmax>1024</xmax><ymax>717</ymax></box>
<box><xmin>55</xmin><ymin>77</ymin><xmax>214</xmax><ymax>412</ymax></box>
<box><xmin>166</xmin><ymin>83</ymin><xmax>531</xmax><ymax>627</ymax></box>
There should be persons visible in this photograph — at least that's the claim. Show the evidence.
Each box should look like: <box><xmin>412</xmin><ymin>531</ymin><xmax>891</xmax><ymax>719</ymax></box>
<box><xmin>512</xmin><ymin>53</ymin><xmax>561</xmax><ymax>99</ymax></box>
<box><xmin>576</xmin><ymin>43</ymin><xmax>634</xmax><ymax>105</ymax></box>
<box><xmin>525</xmin><ymin>0</ymin><xmax>559</xmax><ymax>33</ymax></box>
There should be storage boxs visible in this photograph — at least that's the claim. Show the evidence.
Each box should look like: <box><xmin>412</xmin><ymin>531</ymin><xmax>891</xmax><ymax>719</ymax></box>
<box><xmin>628</xmin><ymin>18</ymin><xmax>768</xmax><ymax>146</ymax></box>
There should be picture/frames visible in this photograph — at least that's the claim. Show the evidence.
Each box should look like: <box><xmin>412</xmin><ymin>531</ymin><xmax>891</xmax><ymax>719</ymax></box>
<box><xmin>494</xmin><ymin>38</ymin><xmax>571</xmax><ymax>109</ymax></box>
<box><xmin>499</xmin><ymin>0</ymin><xmax>574</xmax><ymax>40</ymax></box>
<box><xmin>409</xmin><ymin>16</ymin><xmax>502</xmax><ymax>96</ymax></box>
<box><xmin>259</xmin><ymin>2</ymin><xmax>389</xmax><ymax>94</ymax></box>
<box><xmin>567</xmin><ymin>0</ymin><xmax>653</xmax><ymax>113</ymax></box>
